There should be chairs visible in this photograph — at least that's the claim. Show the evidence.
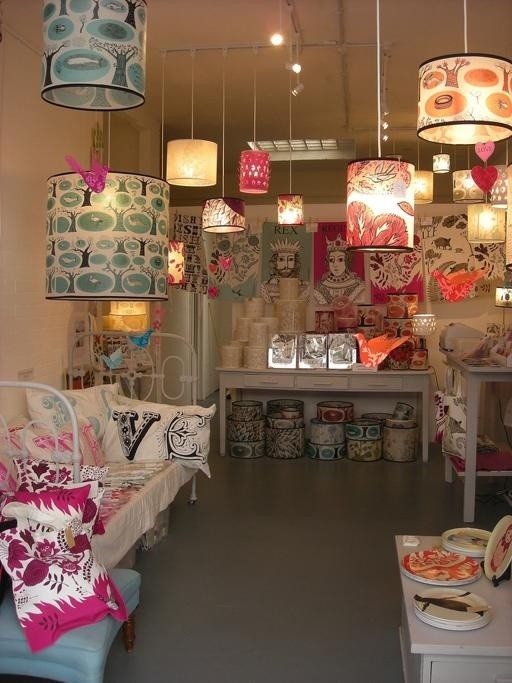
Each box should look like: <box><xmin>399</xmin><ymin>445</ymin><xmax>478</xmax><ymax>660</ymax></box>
<box><xmin>0</xmin><ymin>563</ymin><xmax>142</xmax><ymax>682</ymax></box>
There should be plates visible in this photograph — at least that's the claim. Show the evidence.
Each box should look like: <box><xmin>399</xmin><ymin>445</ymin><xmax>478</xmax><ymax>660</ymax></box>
<box><xmin>413</xmin><ymin>589</ymin><xmax>493</xmax><ymax>630</ymax></box>
<box><xmin>484</xmin><ymin>515</ymin><xmax>512</xmax><ymax>581</ymax></box>
<box><xmin>400</xmin><ymin>550</ymin><xmax>483</xmax><ymax>587</ymax></box>
<box><xmin>440</xmin><ymin>528</ymin><xmax>493</xmax><ymax>556</ymax></box>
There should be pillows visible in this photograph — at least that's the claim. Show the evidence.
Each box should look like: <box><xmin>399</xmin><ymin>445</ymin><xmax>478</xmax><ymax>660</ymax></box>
<box><xmin>98</xmin><ymin>387</ymin><xmax>214</xmax><ymax>478</ymax></box>
<box><xmin>12</xmin><ymin>457</ymin><xmax>106</xmax><ymax>533</ymax></box>
<box><xmin>0</xmin><ymin>521</ymin><xmax>128</xmax><ymax>653</ymax></box>
<box><xmin>0</xmin><ymin>414</ymin><xmax>30</xmax><ymax>497</ymax></box>
<box><xmin>2</xmin><ymin>479</ymin><xmax>98</xmax><ymax>528</ymax></box>
<box><xmin>27</xmin><ymin>417</ymin><xmax>105</xmax><ymax>466</ymax></box>
<box><xmin>27</xmin><ymin>382</ymin><xmax>120</xmax><ymax>442</ymax></box>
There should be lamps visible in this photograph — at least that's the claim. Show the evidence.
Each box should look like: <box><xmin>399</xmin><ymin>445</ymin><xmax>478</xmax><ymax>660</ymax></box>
<box><xmin>110</xmin><ymin>300</ymin><xmax>150</xmax><ymax>331</ymax></box>
<box><xmin>39</xmin><ymin>0</ymin><xmax>147</xmax><ymax>109</ymax></box>
<box><xmin>495</xmin><ymin>286</ymin><xmax>512</xmax><ymax>325</ymax></box>
<box><xmin>413</xmin><ymin>312</ymin><xmax>436</xmax><ymax>348</ymax></box>
<box><xmin>46</xmin><ymin>112</ymin><xmax>169</xmax><ymax>303</ymax></box>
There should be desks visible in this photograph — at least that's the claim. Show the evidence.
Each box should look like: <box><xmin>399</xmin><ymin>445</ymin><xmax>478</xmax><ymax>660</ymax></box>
<box><xmin>395</xmin><ymin>535</ymin><xmax>512</xmax><ymax>683</ymax></box>
<box><xmin>216</xmin><ymin>365</ymin><xmax>435</xmax><ymax>461</ymax></box>
<box><xmin>439</xmin><ymin>345</ymin><xmax>512</xmax><ymax>523</ymax></box>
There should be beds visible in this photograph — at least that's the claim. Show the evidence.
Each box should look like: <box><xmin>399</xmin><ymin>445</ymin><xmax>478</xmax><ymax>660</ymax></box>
<box><xmin>0</xmin><ymin>330</ymin><xmax>198</xmax><ymax>570</ymax></box>
